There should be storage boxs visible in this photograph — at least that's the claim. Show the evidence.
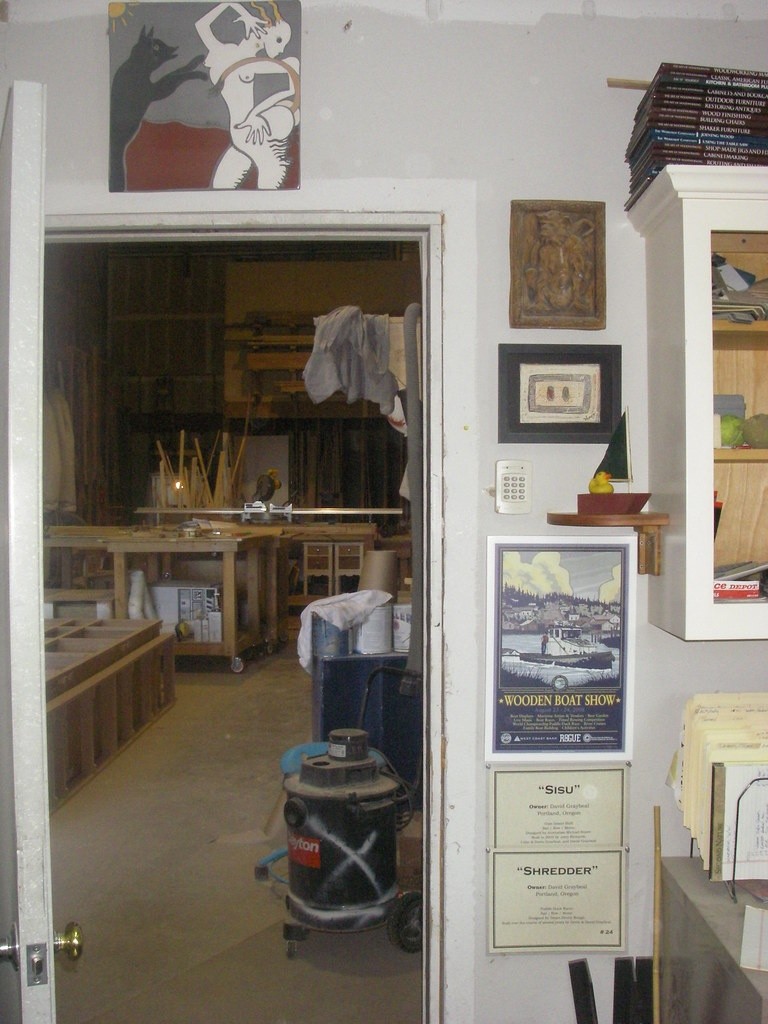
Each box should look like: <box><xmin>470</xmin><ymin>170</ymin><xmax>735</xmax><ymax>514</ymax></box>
<box><xmin>714</xmin><ymin>578</ymin><xmax>761</xmax><ymax>602</ymax></box>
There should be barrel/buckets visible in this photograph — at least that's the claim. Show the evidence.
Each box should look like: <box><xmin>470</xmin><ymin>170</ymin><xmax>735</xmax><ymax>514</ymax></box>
<box><xmin>312</xmin><ymin>611</ymin><xmax>349</xmax><ymax>657</ymax></box>
<box><xmin>355</xmin><ymin>604</ymin><xmax>392</xmax><ymax>654</ymax></box>
<box><xmin>393</xmin><ymin>605</ymin><xmax>412</xmax><ymax>652</ymax></box>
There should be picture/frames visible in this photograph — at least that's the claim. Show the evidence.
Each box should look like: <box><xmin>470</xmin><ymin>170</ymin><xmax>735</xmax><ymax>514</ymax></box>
<box><xmin>498</xmin><ymin>343</ymin><xmax>623</xmax><ymax>446</ymax></box>
<box><xmin>507</xmin><ymin>199</ymin><xmax>607</xmax><ymax>332</ymax></box>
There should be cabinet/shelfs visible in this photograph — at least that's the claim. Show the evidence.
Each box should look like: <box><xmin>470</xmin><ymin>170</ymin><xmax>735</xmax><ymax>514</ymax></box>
<box><xmin>44</xmin><ymin>524</ymin><xmax>377</xmax><ymax>672</ymax></box>
<box><xmin>625</xmin><ymin>164</ymin><xmax>768</xmax><ymax>642</ymax></box>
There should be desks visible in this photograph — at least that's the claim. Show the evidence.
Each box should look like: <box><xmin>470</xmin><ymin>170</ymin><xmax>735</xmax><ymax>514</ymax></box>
<box><xmin>313</xmin><ymin>652</ymin><xmax>423</xmax><ymax>812</ymax></box>
